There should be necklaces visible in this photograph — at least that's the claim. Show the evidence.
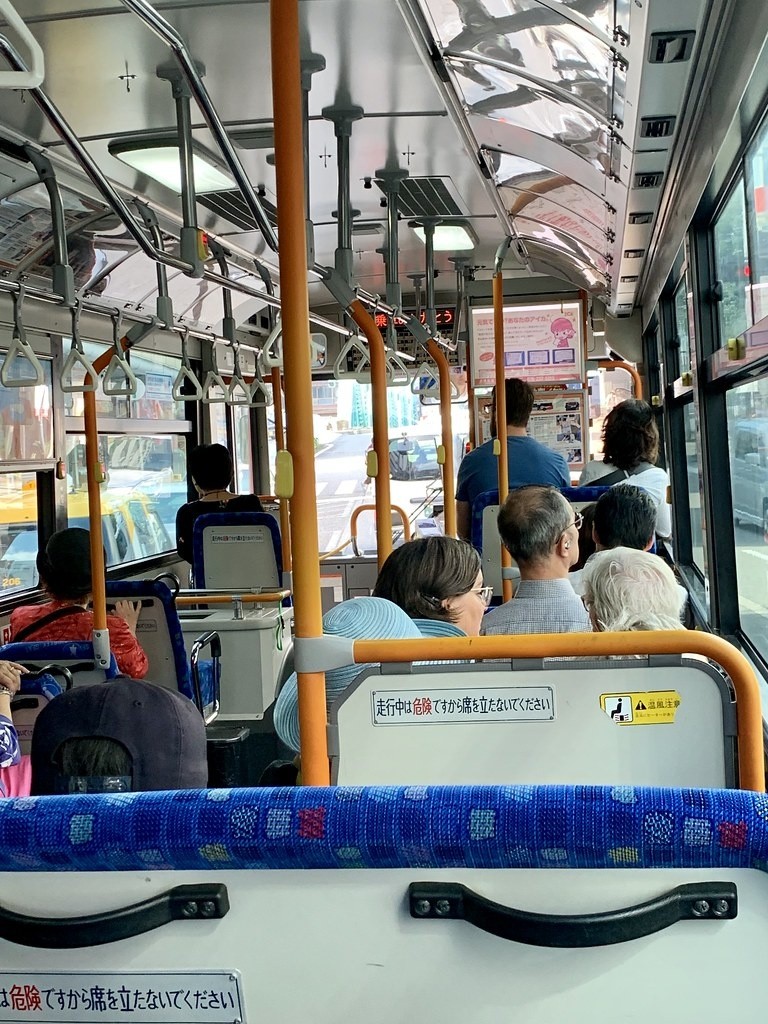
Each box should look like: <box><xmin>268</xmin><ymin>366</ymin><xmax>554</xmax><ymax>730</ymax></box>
<box><xmin>204</xmin><ymin>490</ymin><xmax>228</xmax><ymax>496</ymax></box>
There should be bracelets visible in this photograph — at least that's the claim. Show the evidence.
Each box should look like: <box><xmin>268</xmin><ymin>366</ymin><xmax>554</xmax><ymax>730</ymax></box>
<box><xmin>0</xmin><ymin>687</ymin><xmax>9</xmax><ymax>694</ymax></box>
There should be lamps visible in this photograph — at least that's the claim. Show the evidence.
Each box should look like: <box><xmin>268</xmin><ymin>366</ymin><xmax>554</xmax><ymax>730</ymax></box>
<box><xmin>408</xmin><ymin>219</ymin><xmax>478</xmax><ymax>252</ymax></box>
<box><xmin>109</xmin><ymin>138</ymin><xmax>239</xmax><ymax>196</ymax></box>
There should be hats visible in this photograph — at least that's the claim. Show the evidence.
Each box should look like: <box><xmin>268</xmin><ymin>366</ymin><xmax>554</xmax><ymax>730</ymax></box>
<box><xmin>29</xmin><ymin>674</ymin><xmax>209</xmax><ymax>795</ymax></box>
<box><xmin>273</xmin><ymin>596</ymin><xmax>475</xmax><ymax>760</ymax></box>
<box><xmin>36</xmin><ymin>527</ymin><xmax>107</xmax><ymax>594</ymax></box>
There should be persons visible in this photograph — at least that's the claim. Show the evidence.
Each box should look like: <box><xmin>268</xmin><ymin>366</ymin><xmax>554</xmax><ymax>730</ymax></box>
<box><xmin>578</xmin><ymin>397</ymin><xmax>672</xmax><ymax>539</ymax></box>
<box><xmin>29</xmin><ymin>678</ymin><xmax>209</xmax><ymax>797</ymax></box>
<box><xmin>453</xmin><ymin>378</ymin><xmax>572</xmax><ymax>564</ymax></box>
<box><xmin>0</xmin><ymin>659</ymin><xmax>34</xmax><ymax>797</ymax></box>
<box><xmin>371</xmin><ymin>536</ymin><xmax>496</xmax><ymax>634</ymax></box>
<box><xmin>8</xmin><ymin>527</ymin><xmax>150</xmax><ymax>682</ymax></box>
<box><xmin>252</xmin><ymin>596</ymin><xmax>469</xmax><ymax>790</ymax></box>
<box><xmin>567</xmin><ymin>482</ymin><xmax>658</xmax><ymax>593</ymax></box>
<box><xmin>175</xmin><ymin>444</ymin><xmax>269</xmax><ymax>570</ymax></box>
<box><xmin>478</xmin><ymin>484</ymin><xmax>594</xmax><ymax>665</ymax></box>
<box><xmin>567</xmin><ymin>547</ymin><xmax>709</xmax><ymax>666</ymax></box>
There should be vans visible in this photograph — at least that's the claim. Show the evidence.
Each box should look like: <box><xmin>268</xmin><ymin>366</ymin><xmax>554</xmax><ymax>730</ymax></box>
<box><xmin>729</xmin><ymin>417</ymin><xmax>768</xmax><ymax>535</ymax></box>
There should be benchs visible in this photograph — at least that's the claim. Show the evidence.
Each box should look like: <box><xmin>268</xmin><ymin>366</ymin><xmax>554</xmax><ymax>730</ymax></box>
<box><xmin>324</xmin><ymin>630</ymin><xmax>766</xmax><ymax>794</ymax></box>
<box><xmin>0</xmin><ymin>783</ymin><xmax>768</xmax><ymax>1023</ymax></box>
<box><xmin>471</xmin><ymin>485</ymin><xmax>656</xmax><ymax>609</ymax></box>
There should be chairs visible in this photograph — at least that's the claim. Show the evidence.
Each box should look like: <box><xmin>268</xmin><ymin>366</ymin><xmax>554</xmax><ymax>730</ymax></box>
<box><xmin>193</xmin><ymin>511</ymin><xmax>294</xmax><ymax>607</ymax></box>
<box><xmin>0</xmin><ymin>642</ymin><xmax>121</xmax><ymax>756</ymax></box>
<box><xmin>88</xmin><ymin>579</ymin><xmax>222</xmax><ymax>727</ymax></box>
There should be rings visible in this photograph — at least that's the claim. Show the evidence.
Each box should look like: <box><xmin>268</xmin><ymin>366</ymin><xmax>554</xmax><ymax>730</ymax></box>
<box><xmin>10</xmin><ymin>667</ymin><xmax>14</xmax><ymax>672</ymax></box>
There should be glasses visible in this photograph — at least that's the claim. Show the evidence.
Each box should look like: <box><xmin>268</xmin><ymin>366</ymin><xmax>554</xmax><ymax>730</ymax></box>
<box><xmin>580</xmin><ymin>594</ymin><xmax>595</xmax><ymax>612</ymax></box>
<box><xmin>466</xmin><ymin>584</ymin><xmax>494</xmax><ymax>612</ymax></box>
<box><xmin>556</xmin><ymin>513</ymin><xmax>584</xmax><ymax>546</ymax></box>
<box><xmin>484</xmin><ymin>403</ymin><xmax>496</xmax><ymax>414</ymax></box>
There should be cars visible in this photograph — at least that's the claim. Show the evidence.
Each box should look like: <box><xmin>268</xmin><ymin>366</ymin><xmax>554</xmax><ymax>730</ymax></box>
<box><xmin>0</xmin><ymin>471</ymin><xmax>185</xmax><ymax>601</ymax></box>
